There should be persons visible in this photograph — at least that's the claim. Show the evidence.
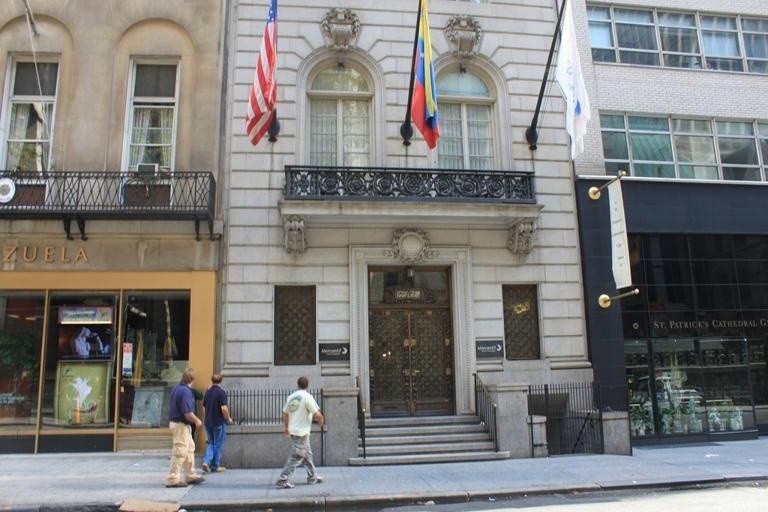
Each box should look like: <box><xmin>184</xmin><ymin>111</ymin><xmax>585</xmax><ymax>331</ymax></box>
<box><xmin>272</xmin><ymin>375</ymin><xmax>326</xmax><ymax>490</ymax></box>
<box><xmin>164</xmin><ymin>367</ymin><xmax>207</xmax><ymax>489</ymax></box>
<box><xmin>201</xmin><ymin>372</ymin><xmax>234</xmax><ymax>474</ymax></box>
<box><xmin>74</xmin><ymin>326</ymin><xmax>92</xmax><ymax>358</ymax></box>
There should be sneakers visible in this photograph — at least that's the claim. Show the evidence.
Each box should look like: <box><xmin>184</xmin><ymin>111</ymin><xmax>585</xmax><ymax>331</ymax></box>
<box><xmin>307</xmin><ymin>477</ymin><xmax>323</xmax><ymax>484</ymax></box>
<box><xmin>217</xmin><ymin>466</ymin><xmax>225</xmax><ymax>473</ymax></box>
<box><xmin>166</xmin><ymin>481</ymin><xmax>187</xmax><ymax>489</ymax></box>
<box><xmin>275</xmin><ymin>481</ymin><xmax>295</xmax><ymax>489</ymax></box>
<box><xmin>203</xmin><ymin>462</ymin><xmax>212</xmax><ymax>472</ymax></box>
<box><xmin>183</xmin><ymin>476</ymin><xmax>205</xmax><ymax>484</ymax></box>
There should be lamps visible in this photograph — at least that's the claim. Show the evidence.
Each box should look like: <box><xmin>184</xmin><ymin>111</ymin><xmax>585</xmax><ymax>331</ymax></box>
<box><xmin>405</xmin><ymin>263</ymin><xmax>415</xmax><ymax>284</ymax></box>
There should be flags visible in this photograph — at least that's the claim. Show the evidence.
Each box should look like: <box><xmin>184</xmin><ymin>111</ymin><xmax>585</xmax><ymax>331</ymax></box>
<box><xmin>552</xmin><ymin>0</ymin><xmax>594</xmax><ymax>162</ymax></box>
<box><xmin>243</xmin><ymin>0</ymin><xmax>280</xmax><ymax>145</ymax></box>
<box><xmin>604</xmin><ymin>177</ymin><xmax>634</xmax><ymax>292</ymax></box>
<box><xmin>408</xmin><ymin>1</ymin><xmax>443</xmax><ymax>151</ymax></box>
